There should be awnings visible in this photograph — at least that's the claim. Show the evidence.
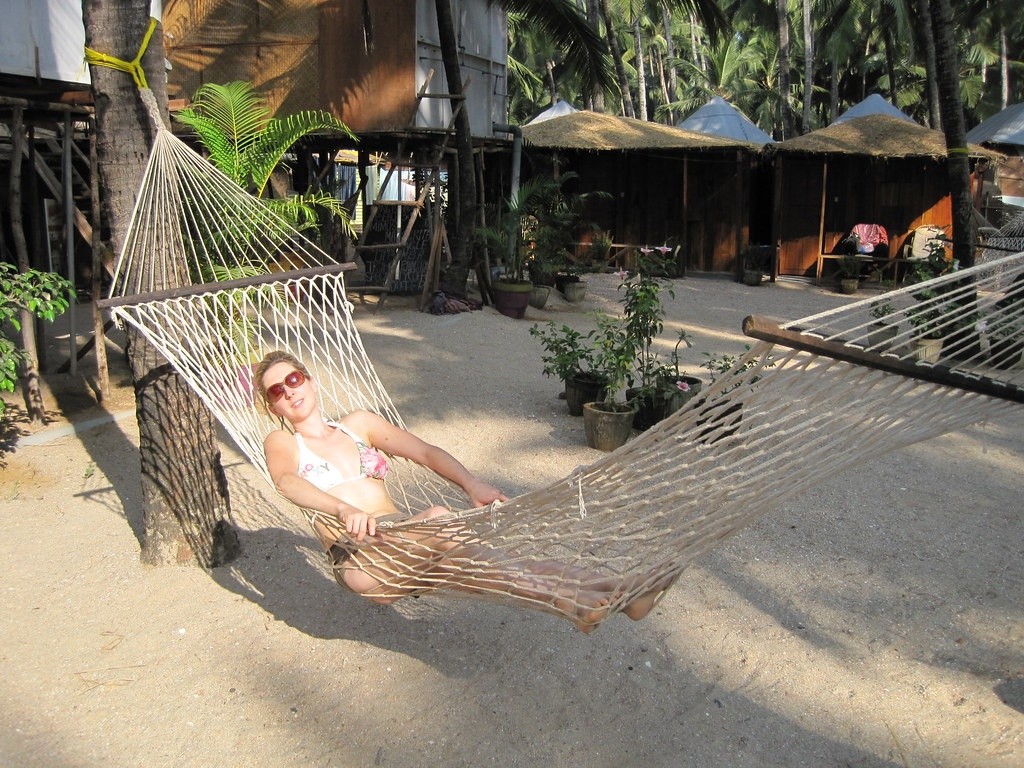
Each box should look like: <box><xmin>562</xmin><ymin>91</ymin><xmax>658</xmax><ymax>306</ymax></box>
<box><xmin>522</xmin><ymin>93</ymin><xmax>1024</xmax><ymax>156</ymax></box>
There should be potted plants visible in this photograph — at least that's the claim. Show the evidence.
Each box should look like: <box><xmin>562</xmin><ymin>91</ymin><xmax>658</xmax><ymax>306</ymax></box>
<box><xmin>561</xmin><ymin>252</ymin><xmax>587</xmax><ymax>302</ymax></box>
<box><xmin>171</xmin><ymin>80</ymin><xmax>359</xmax><ymax>409</ymax></box>
<box><xmin>947</xmin><ymin>304</ymin><xmax>979</xmax><ymax>361</ymax></box>
<box><xmin>582</xmin><ymin>309</ymin><xmax>638</xmax><ymax>451</ymax></box>
<box><xmin>742</xmin><ymin>243</ymin><xmax>770</xmax><ymax>283</ymax></box>
<box><xmin>985</xmin><ymin>300</ymin><xmax>1024</xmax><ymax>369</ymax></box>
<box><xmin>530</xmin><ymin>253</ymin><xmax>555</xmax><ymax>310</ymax></box>
<box><xmin>839</xmin><ymin>257</ymin><xmax>860</xmax><ymax>295</ymax></box>
<box><xmin>905</xmin><ymin>292</ymin><xmax>947</xmax><ymax>364</ymax></box>
<box><xmin>477</xmin><ymin>176</ymin><xmax>562</xmax><ymax>319</ymax></box>
<box><xmin>696</xmin><ymin>345</ymin><xmax>764</xmax><ymax>441</ymax></box>
<box><xmin>867</xmin><ymin>298</ymin><xmax>899</xmax><ymax>351</ymax></box>
<box><xmin>658</xmin><ymin>329</ymin><xmax>702</xmax><ymax>420</ymax></box>
<box><xmin>527</xmin><ymin>321</ymin><xmax>624</xmax><ymax>413</ymax></box>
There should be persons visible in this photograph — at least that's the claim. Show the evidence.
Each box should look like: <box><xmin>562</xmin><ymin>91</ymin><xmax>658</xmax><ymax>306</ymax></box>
<box><xmin>253</xmin><ymin>350</ymin><xmax>685</xmax><ymax>635</ymax></box>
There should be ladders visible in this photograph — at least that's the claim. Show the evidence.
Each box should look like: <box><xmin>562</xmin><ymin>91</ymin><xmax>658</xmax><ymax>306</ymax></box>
<box><xmin>329</xmin><ymin>70</ymin><xmax>471</xmax><ymax>314</ymax></box>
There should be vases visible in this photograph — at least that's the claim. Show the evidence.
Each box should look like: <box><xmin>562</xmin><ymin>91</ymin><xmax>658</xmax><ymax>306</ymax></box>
<box><xmin>628</xmin><ymin>386</ymin><xmax>665</xmax><ymax>430</ymax></box>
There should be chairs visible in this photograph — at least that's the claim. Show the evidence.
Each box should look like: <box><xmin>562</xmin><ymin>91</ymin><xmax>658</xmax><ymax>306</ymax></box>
<box><xmin>847</xmin><ymin>224</ymin><xmax>889</xmax><ymax>278</ymax></box>
<box><xmin>592</xmin><ymin>232</ymin><xmax>619</xmax><ymax>273</ymax></box>
<box><xmin>904</xmin><ymin>225</ymin><xmax>946</xmax><ymax>282</ymax></box>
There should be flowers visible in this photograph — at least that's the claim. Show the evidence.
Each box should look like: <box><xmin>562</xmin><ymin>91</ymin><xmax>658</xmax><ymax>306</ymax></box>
<box><xmin>626</xmin><ymin>243</ymin><xmax>672</xmax><ymax>382</ymax></box>
